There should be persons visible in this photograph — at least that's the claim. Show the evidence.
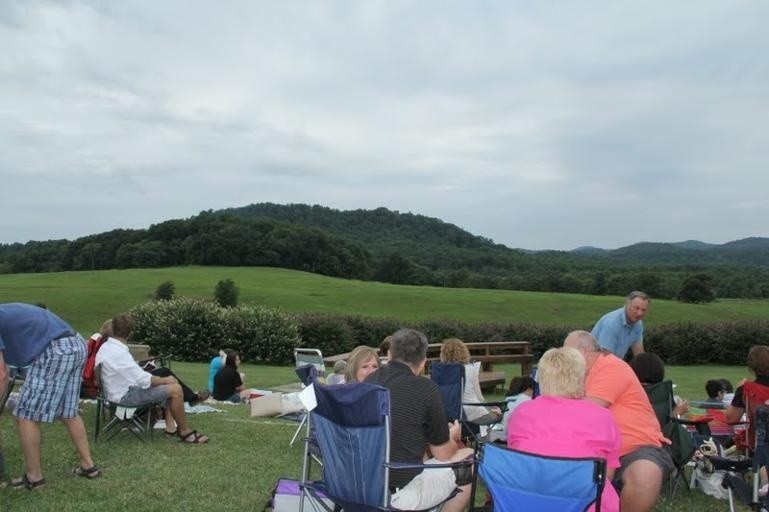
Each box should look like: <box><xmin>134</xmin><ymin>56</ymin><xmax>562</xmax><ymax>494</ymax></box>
<box><xmin>324</xmin><ymin>291</ymin><xmax>768</xmax><ymax>512</ymax></box>
<box><xmin>79</xmin><ymin>318</ymin><xmax>209</xmax><ymax>406</ymax></box>
<box><xmin>0</xmin><ymin>301</ymin><xmax>104</xmax><ymax>490</ymax></box>
<box><xmin>207</xmin><ymin>347</ymin><xmax>245</xmax><ymax>397</ymax></box>
<box><xmin>212</xmin><ymin>351</ymin><xmax>252</xmax><ymax>402</ymax></box>
<box><xmin>93</xmin><ymin>313</ymin><xmax>209</xmax><ymax>444</ymax></box>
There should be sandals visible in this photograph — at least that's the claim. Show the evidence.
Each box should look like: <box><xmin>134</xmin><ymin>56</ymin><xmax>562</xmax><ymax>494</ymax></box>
<box><xmin>164</xmin><ymin>426</ymin><xmax>209</xmax><ymax>443</ymax></box>
<box><xmin>72</xmin><ymin>464</ymin><xmax>100</xmax><ymax>478</ymax></box>
<box><xmin>12</xmin><ymin>474</ymin><xmax>45</xmax><ymax>491</ymax></box>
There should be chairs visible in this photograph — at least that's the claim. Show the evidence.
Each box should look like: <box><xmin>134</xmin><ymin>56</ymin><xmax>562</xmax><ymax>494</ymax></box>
<box><xmin>94</xmin><ymin>353</ymin><xmax>172</xmax><ymax>442</ymax></box>
<box><xmin>289</xmin><ymin>348</ymin><xmax>325</xmax><ymax>448</ymax></box>
<box><xmin>630</xmin><ymin>380</ymin><xmax>769</xmax><ymax>512</ymax></box>
<box><xmin>431</xmin><ymin>363</ymin><xmax>508</xmax><ymax>444</ymax></box>
<box><xmin>477</xmin><ymin>443</ymin><xmax>606</xmax><ymax>512</ymax></box>
<box><xmin>294</xmin><ymin>365</ymin><xmax>478</xmax><ymax>512</ymax></box>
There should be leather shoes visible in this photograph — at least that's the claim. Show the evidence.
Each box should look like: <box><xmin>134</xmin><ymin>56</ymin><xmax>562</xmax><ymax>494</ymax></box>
<box><xmin>190</xmin><ymin>391</ymin><xmax>209</xmax><ymax>406</ymax></box>
